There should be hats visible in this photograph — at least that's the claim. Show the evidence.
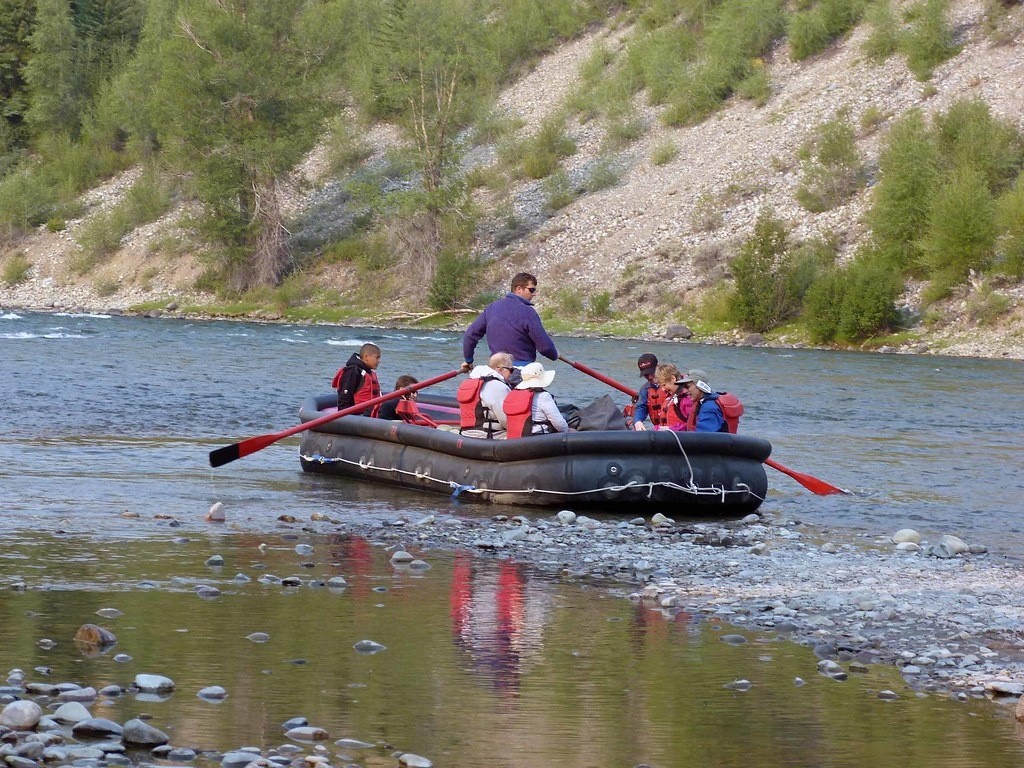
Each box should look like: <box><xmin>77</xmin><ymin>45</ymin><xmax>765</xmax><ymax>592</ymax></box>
<box><xmin>638</xmin><ymin>354</ymin><xmax>658</xmax><ymax>377</ymax></box>
<box><xmin>674</xmin><ymin>369</ymin><xmax>710</xmax><ymax>389</ymax></box>
<box><xmin>515</xmin><ymin>362</ymin><xmax>556</xmax><ymax>390</ymax></box>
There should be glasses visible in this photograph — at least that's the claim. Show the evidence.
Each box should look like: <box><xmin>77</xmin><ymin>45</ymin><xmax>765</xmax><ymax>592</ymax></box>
<box><xmin>498</xmin><ymin>367</ymin><xmax>514</xmax><ymax>373</ymax></box>
<box><xmin>412</xmin><ymin>390</ymin><xmax>418</xmax><ymax>395</ymax></box>
<box><xmin>515</xmin><ymin>284</ymin><xmax>536</xmax><ymax>293</ymax></box>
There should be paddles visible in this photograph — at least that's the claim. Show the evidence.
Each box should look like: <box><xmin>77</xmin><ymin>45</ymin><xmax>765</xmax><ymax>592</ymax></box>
<box><xmin>209</xmin><ymin>364</ymin><xmax>470</xmax><ymax>468</ymax></box>
<box><xmin>558</xmin><ymin>356</ymin><xmax>840</xmax><ymax>497</ymax></box>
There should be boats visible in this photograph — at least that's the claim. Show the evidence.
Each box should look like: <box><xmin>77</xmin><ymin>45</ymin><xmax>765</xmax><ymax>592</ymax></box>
<box><xmin>298</xmin><ymin>390</ymin><xmax>772</xmax><ymax>518</ymax></box>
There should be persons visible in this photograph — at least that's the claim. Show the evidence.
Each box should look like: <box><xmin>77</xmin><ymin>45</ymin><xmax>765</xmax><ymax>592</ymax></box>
<box><xmin>674</xmin><ymin>370</ymin><xmax>730</xmax><ymax>433</ymax></box>
<box><xmin>331</xmin><ymin>344</ymin><xmax>383</xmax><ymax>417</ymax></box>
<box><xmin>461</xmin><ymin>272</ymin><xmax>562</xmax><ymax>387</ymax></box>
<box><xmin>632</xmin><ymin>354</ymin><xmax>670</xmax><ymax>432</ymax></box>
<box><xmin>369</xmin><ymin>374</ymin><xmax>419</xmax><ymax>420</ymax></box>
<box><xmin>456</xmin><ymin>352</ymin><xmax>514</xmax><ymax>441</ymax></box>
<box><xmin>502</xmin><ymin>362</ymin><xmax>577</xmax><ymax>439</ymax></box>
<box><xmin>653</xmin><ymin>363</ymin><xmax>695</xmax><ymax>432</ymax></box>
